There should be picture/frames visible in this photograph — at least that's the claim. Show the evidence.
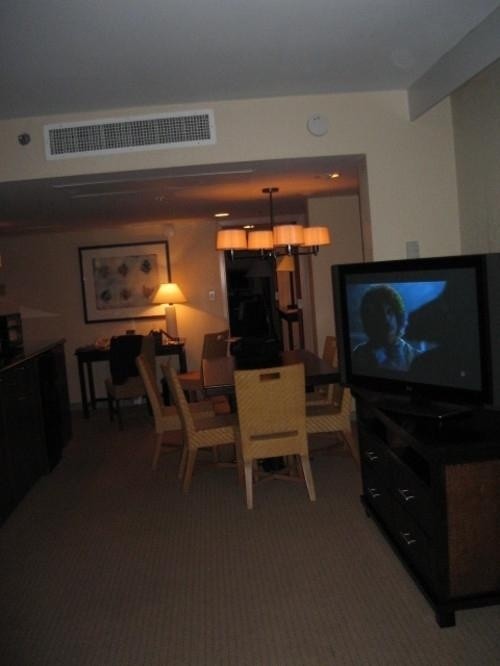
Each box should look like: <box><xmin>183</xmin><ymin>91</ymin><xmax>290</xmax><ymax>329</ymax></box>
<box><xmin>79</xmin><ymin>241</ymin><xmax>174</xmax><ymax>324</ymax></box>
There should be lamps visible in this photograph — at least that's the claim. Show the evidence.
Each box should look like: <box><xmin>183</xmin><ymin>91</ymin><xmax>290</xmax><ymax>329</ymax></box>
<box><xmin>153</xmin><ymin>283</ymin><xmax>187</xmax><ymax>340</ymax></box>
<box><xmin>216</xmin><ymin>188</ymin><xmax>331</xmax><ymax>262</ymax></box>
<box><xmin>0</xmin><ymin>283</ymin><xmax>22</xmax><ymax>358</ymax></box>
<box><xmin>162</xmin><ymin>224</ymin><xmax>175</xmax><ymax>239</ymax></box>
<box><xmin>276</xmin><ymin>255</ymin><xmax>297</xmax><ymax>309</ymax></box>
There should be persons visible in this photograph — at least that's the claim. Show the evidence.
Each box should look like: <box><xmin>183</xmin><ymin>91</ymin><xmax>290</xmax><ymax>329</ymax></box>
<box><xmin>353</xmin><ymin>284</ymin><xmax>419</xmax><ymax>372</ymax></box>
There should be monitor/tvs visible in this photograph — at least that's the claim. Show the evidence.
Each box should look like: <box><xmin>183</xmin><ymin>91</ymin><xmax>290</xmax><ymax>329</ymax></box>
<box><xmin>330</xmin><ymin>251</ymin><xmax>499</xmax><ymax>419</ymax></box>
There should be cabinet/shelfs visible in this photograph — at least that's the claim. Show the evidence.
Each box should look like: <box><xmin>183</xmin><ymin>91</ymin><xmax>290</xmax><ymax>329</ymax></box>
<box><xmin>0</xmin><ymin>337</ymin><xmax>73</xmax><ymax>526</ymax></box>
<box><xmin>349</xmin><ymin>388</ymin><xmax>499</xmax><ymax>628</ymax></box>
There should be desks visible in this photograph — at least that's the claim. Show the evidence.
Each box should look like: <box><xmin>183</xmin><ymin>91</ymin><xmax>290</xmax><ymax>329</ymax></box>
<box><xmin>200</xmin><ymin>346</ymin><xmax>338</xmax><ymax>474</ymax></box>
<box><xmin>75</xmin><ymin>338</ymin><xmax>190</xmax><ymax>419</ymax></box>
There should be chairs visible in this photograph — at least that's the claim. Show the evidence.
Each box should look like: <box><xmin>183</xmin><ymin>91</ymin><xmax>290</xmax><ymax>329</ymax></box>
<box><xmin>232</xmin><ymin>361</ymin><xmax>318</xmax><ymax>510</ymax></box>
<box><xmin>288</xmin><ymin>335</ymin><xmax>338</xmax><ymax>468</ymax></box>
<box><xmin>167</xmin><ymin>329</ymin><xmax>232</xmax><ymax>406</ymax></box>
<box><xmin>137</xmin><ymin>353</ymin><xmax>217</xmax><ymax>471</ymax></box>
<box><xmin>163</xmin><ymin>366</ymin><xmax>259</xmax><ymax>492</ymax></box>
<box><xmin>305</xmin><ymin>383</ymin><xmax>352</xmax><ymax>469</ymax></box>
